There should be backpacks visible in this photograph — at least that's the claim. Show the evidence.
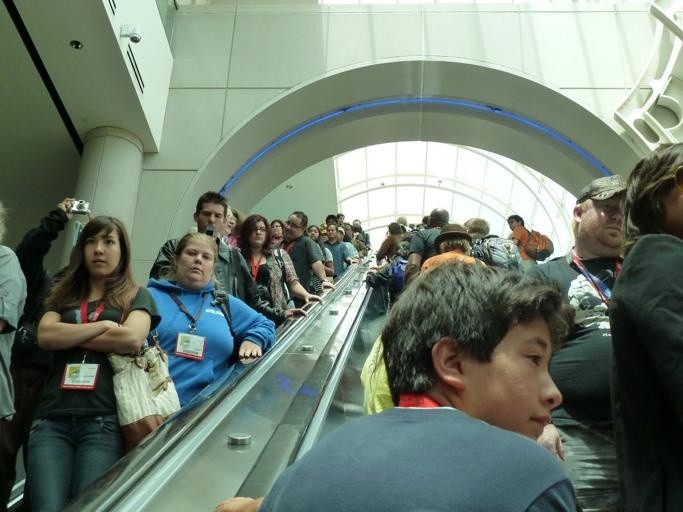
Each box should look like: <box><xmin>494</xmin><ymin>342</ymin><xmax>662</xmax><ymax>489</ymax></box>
<box><xmin>519</xmin><ymin>225</ymin><xmax>554</xmax><ymax>261</ymax></box>
<box><xmin>471</xmin><ymin>235</ymin><xmax>520</xmax><ymax>267</ymax></box>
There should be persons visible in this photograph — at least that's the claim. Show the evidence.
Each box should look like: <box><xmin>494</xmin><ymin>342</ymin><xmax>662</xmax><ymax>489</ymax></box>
<box><xmin>259</xmin><ymin>259</ymin><xmax>578</xmax><ymax>512</ymax></box>
<box><xmin>11</xmin><ymin>198</ymin><xmax>70</xmax><ymax>499</ymax></box>
<box><xmin>27</xmin><ymin>216</ymin><xmax>162</xmax><ymax>512</ymax></box>
<box><xmin>534</xmin><ymin>173</ymin><xmax>626</xmax><ymax>512</ymax></box>
<box><xmin>151</xmin><ymin>191</ymin><xmax>304</xmax><ymax>323</ymax></box>
<box><xmin>1</xmin><ymin>205</ymin><xmax>27</xmax><ymax>512</ymax></box>
<box><xmin>610</xmin><ymin>143</ymin><xmax>682</xmax><ymax>512</ymax></box>
<box><xmin>359</xmin><ymin>332</ymin><xmax>395</xmax><ymax>417</ymax></box>
<box><xmin>220</xmin><ymin>205</ymin><xmax>538</xmax><ymax>320</ymax></box>
<box><xmin>145</xmin><ymin>231</ymin><xmax>276</xmax><ymax>406</ymax></box>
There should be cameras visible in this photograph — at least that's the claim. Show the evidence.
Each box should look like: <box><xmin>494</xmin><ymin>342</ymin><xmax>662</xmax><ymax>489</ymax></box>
<box><xmin>70</xmin><ymin>200</ymin><xmax>90</xmax><ymax>214</ymax></box>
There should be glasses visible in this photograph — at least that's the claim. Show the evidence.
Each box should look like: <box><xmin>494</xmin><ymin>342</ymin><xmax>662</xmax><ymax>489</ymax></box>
<box><xmin>283</xmin><ymin>221</ymin><xmax>305</xmax><ymax>228</ymax></box>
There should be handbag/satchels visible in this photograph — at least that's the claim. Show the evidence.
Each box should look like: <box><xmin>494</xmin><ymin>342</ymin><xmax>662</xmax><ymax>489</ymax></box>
<box><xmin>107</xmin><ymin>348</ymin><xmax>181</xmax><ymax>452</ymax></box>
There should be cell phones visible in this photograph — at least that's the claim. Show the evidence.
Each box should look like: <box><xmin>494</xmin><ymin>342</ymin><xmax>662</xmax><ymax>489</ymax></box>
<box><xmin>205</xmin><ymin>226</ymin><xmax>215</xmax><ymax>237</ymax></box>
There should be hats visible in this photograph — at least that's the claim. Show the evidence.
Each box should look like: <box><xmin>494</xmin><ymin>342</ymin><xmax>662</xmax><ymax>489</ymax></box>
<box><xmin>574</xmin><ymin>175</ymin><xmax>628</xmax><ymax>203</ymax></box>
<box><xmin>434</xmin><ymin>224</ymin><xmax>472</xmax><ymax>253</ymax></box>
<box><xmin>336</xmin><ymin>227</ymin><xmax>344</xmax><ymax>234</ymax></box>
